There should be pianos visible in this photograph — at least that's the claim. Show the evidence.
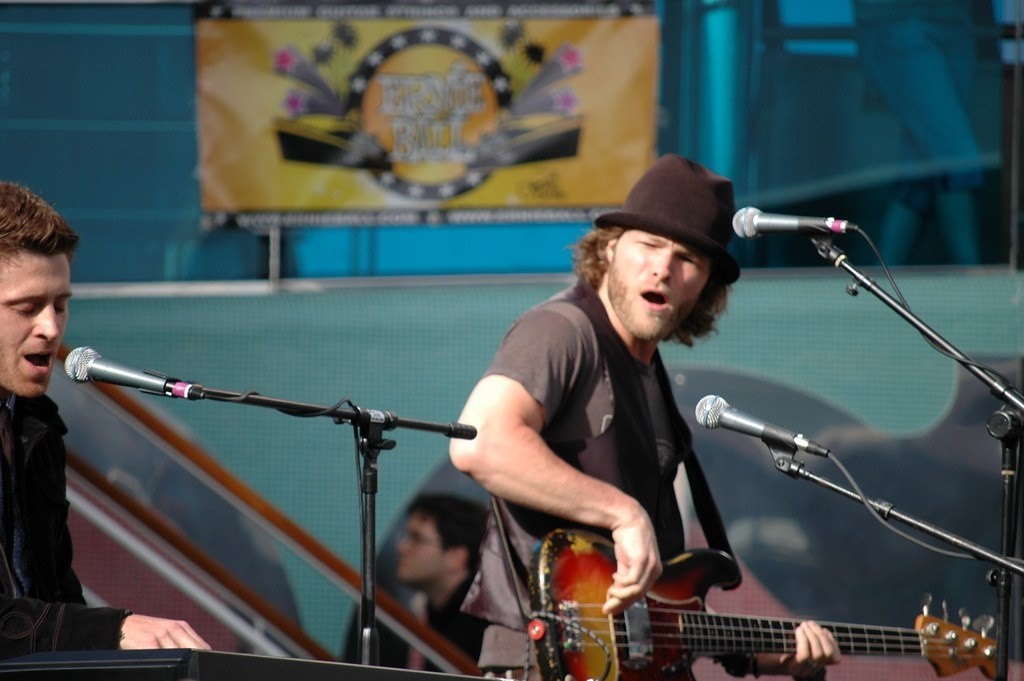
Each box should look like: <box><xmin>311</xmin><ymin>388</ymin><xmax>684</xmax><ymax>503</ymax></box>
<box><xmin>0</xmin><ymin>647</ymin><xmax>511</xmax><ymax>680</ymax></box>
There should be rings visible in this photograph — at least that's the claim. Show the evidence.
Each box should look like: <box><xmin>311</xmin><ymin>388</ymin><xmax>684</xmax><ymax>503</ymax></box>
<box><xmin>806</xmin><ymin>661</ymin><xmax>817</xmax><ymax>669</ymax></box>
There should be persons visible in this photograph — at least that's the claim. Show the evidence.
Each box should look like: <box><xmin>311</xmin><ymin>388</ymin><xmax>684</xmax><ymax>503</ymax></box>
<box><xmin>449</xmin><ymin>155</ymin><xmax>842</xmax><ymax>681</ymax></box>
<box><xmin>395</xmin><ymin>490</ymin><xmax>488</xmax><ymax>681</ymax></box>
<box><xmin>0</xmin><ymin>183</ymin><xmax>211</xmax><ymax>659</ymax></box>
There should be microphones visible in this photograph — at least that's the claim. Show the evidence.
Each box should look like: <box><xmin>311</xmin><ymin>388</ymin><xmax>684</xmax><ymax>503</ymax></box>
<box><xmin>65</xmin><ymin>346</ymin><xmax>209</xmax><ymax>401</ymax></box>
<box><xmin>696</xmin><ymin>394</ymin><xmax>831</xmax><ymax>458</ymax></box>
<box><xmin>732</xmin><ymin>206</ymin><xmax>859</xmax><ymax>240</ymax></box>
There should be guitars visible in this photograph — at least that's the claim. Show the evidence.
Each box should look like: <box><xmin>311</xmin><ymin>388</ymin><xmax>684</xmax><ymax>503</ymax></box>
<box><xmin>530</xmin><ymin>527</ymin><xmax>997</xmax><ymax>680</ymax></box>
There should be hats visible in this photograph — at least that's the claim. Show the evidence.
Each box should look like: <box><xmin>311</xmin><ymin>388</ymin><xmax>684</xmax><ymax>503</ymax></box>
<box><xmin>594</xmin><ymin>153</ymin><xmax>742</xmax><ymax>286</ymax></box>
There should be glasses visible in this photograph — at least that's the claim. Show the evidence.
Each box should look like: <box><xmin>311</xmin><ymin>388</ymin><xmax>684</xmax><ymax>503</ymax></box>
<box><xmin>396</xmin><ymin>528</ymin><xmax>452</xmax><ymax>550</ymax></box>
